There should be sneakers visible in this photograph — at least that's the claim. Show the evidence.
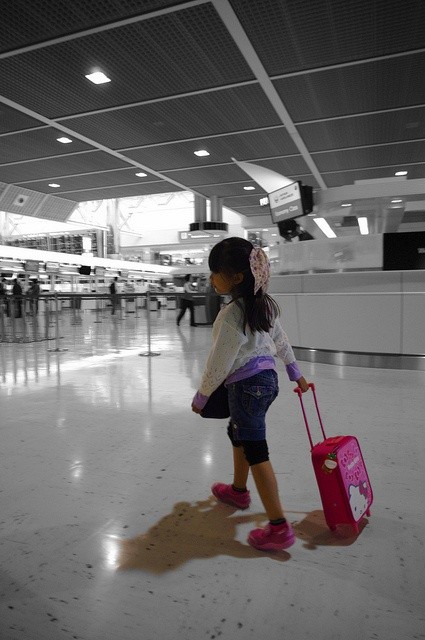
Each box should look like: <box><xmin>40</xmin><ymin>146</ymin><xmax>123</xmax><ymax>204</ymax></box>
<box><xmin>211</xmin><ymin>481</ymin><xmax>251</xmax><ymax>509</ymax></box>
<box><xmin>247</xmin><ymin>522</ymin><xmax>296</xmax><ymax>551</ymax></box>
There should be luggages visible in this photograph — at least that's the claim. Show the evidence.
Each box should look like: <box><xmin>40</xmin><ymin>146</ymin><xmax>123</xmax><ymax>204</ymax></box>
<box><xmin>293</xmin><ymin>383</ymin><xmax>374</xmax><ymax>539</ymax></box>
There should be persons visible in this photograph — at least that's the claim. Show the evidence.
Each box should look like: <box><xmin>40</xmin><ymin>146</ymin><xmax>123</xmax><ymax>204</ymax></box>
<box><xmin>12</xmin><ymin>279</ymin><xmax>23</xmax><ymax>318</ymax></box>
<box><xmin>176</xmin><ymin>273</ymin><xmax>197</xmax><ymax>327</ymax></box>
<box><xmin>28</xmin><ymin>282</ymin><xmax>39</xmax><ymax>313</ymax></box>
<box><xmin>191</xmin><ymin>236</ymin><xmax>309</xmax><ymax>551</ymax></box>
<box><xmin>0</xmin><ymin>278</ymin><xmax>7</xmax><ymax>300</ymax></box>
<box><xmin>110</xmin><ymin>277</ymin><xmax>118</xmax><ymax>315</ymax></box>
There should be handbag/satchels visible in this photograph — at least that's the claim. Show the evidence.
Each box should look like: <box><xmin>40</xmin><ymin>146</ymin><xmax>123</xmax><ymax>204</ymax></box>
<box><xmin>201</xmin><ymin>381</ymin><xmax>231</xmax><ymax>419</ymax></box>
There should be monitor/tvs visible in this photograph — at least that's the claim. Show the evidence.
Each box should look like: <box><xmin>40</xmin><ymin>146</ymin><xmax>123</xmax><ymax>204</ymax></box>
<box><xmin>268</xmin><ymin>181</ymin><xmax>313</xmax><ymax>224</ymax></box>
